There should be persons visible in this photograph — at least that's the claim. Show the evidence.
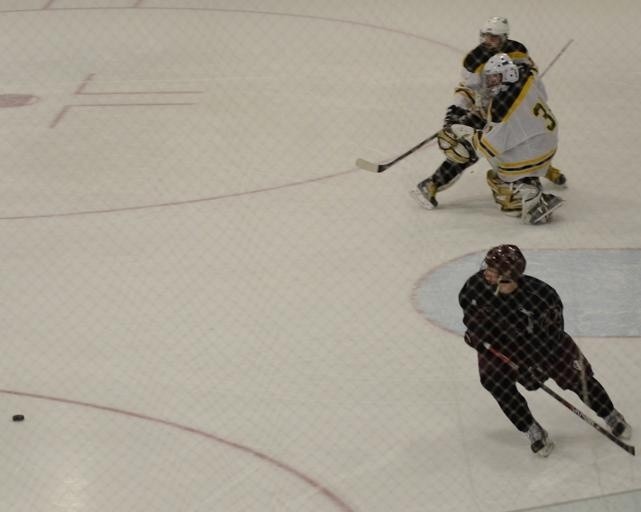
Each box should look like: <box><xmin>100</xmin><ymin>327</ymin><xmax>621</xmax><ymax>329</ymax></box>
<box><xmin>459</xmin><ymin>243</ymin><xmax>628</xmax><ymax>455</ymax></box>
<box><xmin>439</xmin><ymin>50</ymin><xmax>567</xmax><ymax>225</ymax></box>
<box><xmin>417</xmin><ymin>14</ymin><xmax>548</xmax><ymax>207</ymax></box>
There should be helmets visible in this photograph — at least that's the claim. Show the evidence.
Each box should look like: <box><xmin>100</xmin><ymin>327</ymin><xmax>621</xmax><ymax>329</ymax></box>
<box><xmin>480</xmin><ymin>244</ymin><xmax>525</xmax><ymax>280</ymax></box>
<box><xmin>482</xmin><ymin>52</ymin><xmax>520</xmax><ymax>96</ymax></box>
<box><xmin>479</xmin><ymin>17</ymin><xmax>510</xmax><ymax>51</ymax></box>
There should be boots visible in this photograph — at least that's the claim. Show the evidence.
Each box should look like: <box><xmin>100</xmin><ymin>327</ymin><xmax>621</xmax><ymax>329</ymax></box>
<box><xmin>411</xmin><ymin>177</ymin><xmax>439</xmax><ymax>208</ymax></box>
<box><xmin>500</xmin><ymin>204</ymin><xmax>522</xmax><ymax>214</ymax></box>
<box><xmin>553</xmin><ymin>174</ymin><xmax>566</xmax><ymax>186</ymax></box>
<box><xmin>523</xmin><ymin>421</ymin><xmax>550</xmax><ymax>454</ymax></box>
<box><xmin>602</xmin><ymin>410</ymin><xmax>626</xmax><ymax>437</ymax></box>
<box><xmin>524</xmin><ymin>194</ymin><xmax>562</xmax><ymax>223</ymax></box>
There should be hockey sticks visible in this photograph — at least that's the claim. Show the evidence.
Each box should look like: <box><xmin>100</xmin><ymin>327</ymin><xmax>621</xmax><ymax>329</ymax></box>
<box><xmin>355</xmin><ymin>128</ymin><xmax>444</xmax><ymax>173</ymax></box>
